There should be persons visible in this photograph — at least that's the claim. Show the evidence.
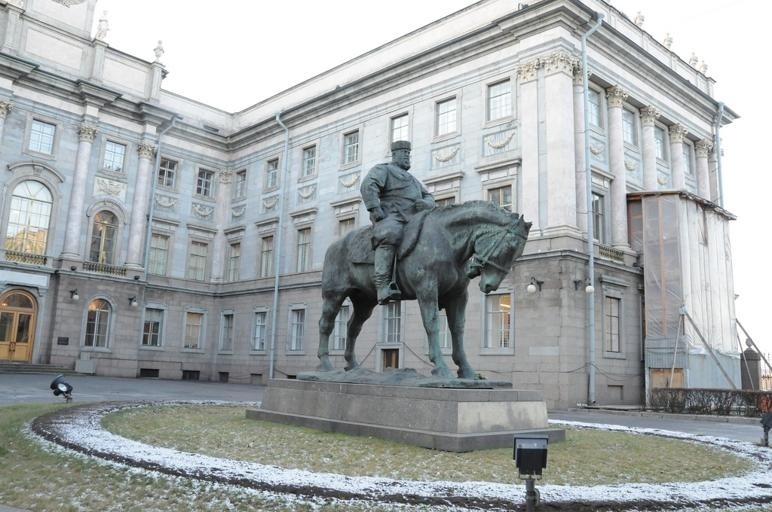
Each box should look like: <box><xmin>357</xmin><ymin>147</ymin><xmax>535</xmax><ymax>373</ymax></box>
<box><xmin>360</xmin><ymin>141</ymin><xmax>436</xmax><ymax>304</ymax></box>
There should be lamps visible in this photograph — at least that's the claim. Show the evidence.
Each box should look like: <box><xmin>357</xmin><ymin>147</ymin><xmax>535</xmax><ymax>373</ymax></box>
<box><xmin>50</xmin><ymin>374</ymin><xmax>73</xmax><ymax>405</ymax></box>
<box><xmin>511</xmin><ymin>431</ymin><xmax>548</xmax><ymax>511</ymax></box>
<box><xmin>574</xmin><ymin>277</ymin><xmax>594</xmax><ymax>294</ymax></box>
<box><xmin>69</xmin><ymin>287</ymin><xmax>80</xmax><ymax>301</ymax></box>
<box><xmin>128</xmin><ymin>296</ymin><xmax>139</xmax><ymax>307</ymax></box>
<box><xmin>525</xmin><ymin>276</ymin><xmax>544</xmax><ymax>293</ymax></box>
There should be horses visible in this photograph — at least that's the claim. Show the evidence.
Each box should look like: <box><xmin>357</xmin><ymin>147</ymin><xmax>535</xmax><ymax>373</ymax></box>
<box><xmin>317</xmin><ymin>199</ymin><xmax>532</xmax><ymax>381</ymax></box>
